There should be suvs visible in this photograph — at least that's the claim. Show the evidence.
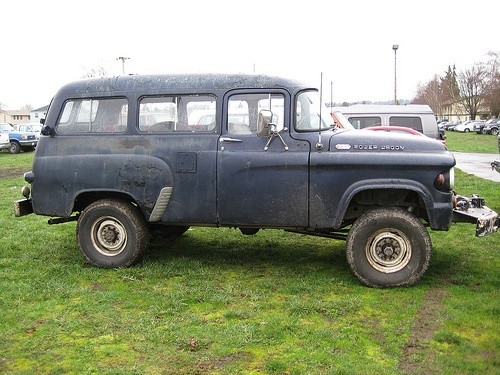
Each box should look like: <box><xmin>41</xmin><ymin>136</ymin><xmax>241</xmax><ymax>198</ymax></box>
<box><xmin>14</xmin><ymin>74</ymin><xmax>500</xmax><ymax>290</ymax></box>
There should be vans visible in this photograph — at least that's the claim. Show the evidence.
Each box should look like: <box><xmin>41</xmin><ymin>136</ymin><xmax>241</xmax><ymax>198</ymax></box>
<box><xmin>328</xmin><ymin>103</ymin><xmax>447</xmax><ymax>138</ymax></box>
<box><xmin>185</xmin><ymin>105</ymin><xmax>289</xmax><ymax>135</ymax></box>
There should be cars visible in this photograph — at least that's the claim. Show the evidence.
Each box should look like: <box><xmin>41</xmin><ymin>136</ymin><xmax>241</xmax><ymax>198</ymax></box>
<box><xmin>361</xmin><ymin>126</ymin><xmax>447</xmax><ymax>149</ymax></box>
<box><xmin>12</xmin><ymin>124</ymin><xmax>41</xmax><ymax>141</ymax></box>
<box><xmin>0</xmin><ymin>123</ymin><xmax>38</xmax><ymax>153</ymax></box>
<box><xmin>0</xmin><ymin>130</ymin><xmax>11</xmax><ymax>151</ymax></box>
<box><xmin>436</xmin><ymin>117</ymin><xmax>500</xmax><ymax>136</ymax></box>
